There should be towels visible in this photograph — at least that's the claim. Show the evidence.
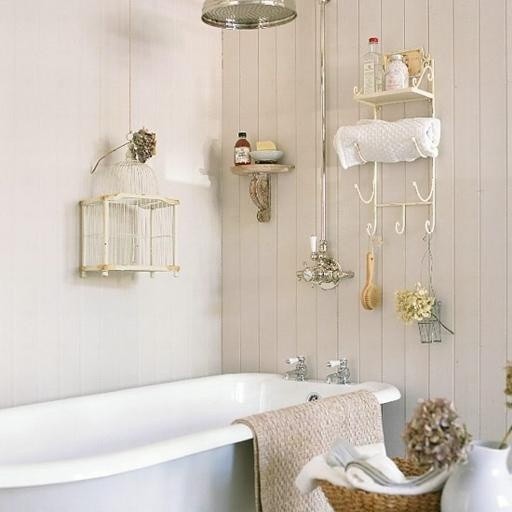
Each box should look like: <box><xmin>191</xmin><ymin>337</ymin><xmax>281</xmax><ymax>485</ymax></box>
<box><xmin>291</xmin><ymin>440</ymin><xmax>454</xmax><ymax>496</ymax></box>
<box><xmin>332</xmin><ymin>117</ymin><xmax>442</xmax><ymax>171</ymax></box>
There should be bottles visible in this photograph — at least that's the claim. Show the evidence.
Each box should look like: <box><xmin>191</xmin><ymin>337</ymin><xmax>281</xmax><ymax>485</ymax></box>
<box><xmin>234</xmin><ymin>131</ymin><xmax>251</xmax><ymax>165</ymax></box>
<box><xmin>361</xmin><ymin>37</ymin><xmax>410</xmax><ymax>94</ymax></box>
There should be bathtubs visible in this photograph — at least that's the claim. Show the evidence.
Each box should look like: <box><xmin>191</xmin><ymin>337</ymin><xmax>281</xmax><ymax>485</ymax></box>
<box><xmin>0</xmin><ymin>372</ymin><xmax>402</xmax><ymax>512</ymax></box>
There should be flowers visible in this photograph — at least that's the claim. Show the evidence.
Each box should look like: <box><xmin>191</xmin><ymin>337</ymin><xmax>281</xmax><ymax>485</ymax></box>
<box><xmin>403</xmin><ymin>394</ymin><xmax>472</xmax><ymax>467</ymax></box>
<box><xmin>394</xmin><ymin>281</ymin><xmax>455</xmax><ymax>336</ymax></box>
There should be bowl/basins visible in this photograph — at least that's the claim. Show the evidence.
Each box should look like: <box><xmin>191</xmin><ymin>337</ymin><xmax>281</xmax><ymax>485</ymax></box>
<box><xmin>248</xmin><ymin>150</ymin><xmax>284</xmax><ymax>161</ymax></box>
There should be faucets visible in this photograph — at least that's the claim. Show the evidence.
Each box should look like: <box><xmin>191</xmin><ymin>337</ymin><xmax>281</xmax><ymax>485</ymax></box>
<box><xmin>282</xmin><ymin>356</ymin><xmax>308</xmax><ymax>381</ymax></box>
<box><xmin>324</xmin><ymin>358</ymin><xmax>351</xmax><ymax>384</ymax></box>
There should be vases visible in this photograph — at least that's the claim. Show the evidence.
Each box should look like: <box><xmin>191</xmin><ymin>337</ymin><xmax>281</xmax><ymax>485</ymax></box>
<box><xmin>439</xmin><ymin>440</ymin><xmax>511</xmax><ymax>512</ymax></box>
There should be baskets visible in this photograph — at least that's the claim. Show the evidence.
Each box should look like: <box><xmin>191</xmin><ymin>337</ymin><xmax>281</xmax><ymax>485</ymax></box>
<box><xmin>315</xmin><ymin>457</ymin><xmax>442</xmax><ymax>511</ymax></box>
